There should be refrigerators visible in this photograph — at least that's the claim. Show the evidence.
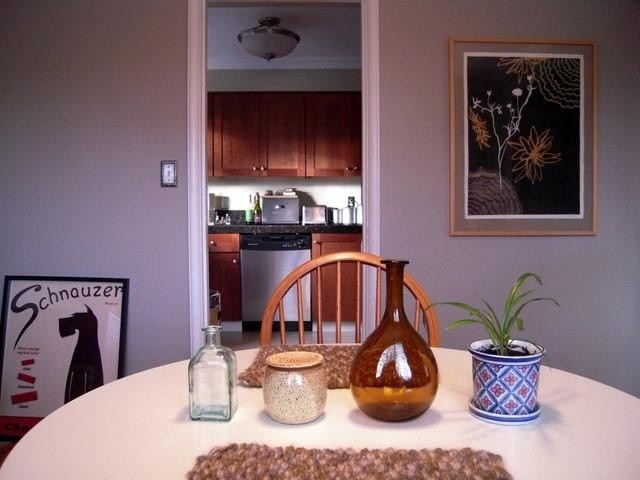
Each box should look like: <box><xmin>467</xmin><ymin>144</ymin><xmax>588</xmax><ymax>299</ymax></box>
<box><xmin>241</xmin><ymin>234</ymin><xmax>311</xmax><ymax>321</ymax></box>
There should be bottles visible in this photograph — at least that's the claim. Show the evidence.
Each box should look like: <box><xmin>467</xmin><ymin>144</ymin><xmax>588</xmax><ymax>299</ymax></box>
<box><xmin>182</xmin><ymin>323</ymin><xmax>240</xmax><ymax>418</ymax></box>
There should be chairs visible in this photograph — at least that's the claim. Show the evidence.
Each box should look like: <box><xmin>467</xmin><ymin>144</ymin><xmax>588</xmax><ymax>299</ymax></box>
<box><xmin>260</xmin><ymin>250</ymin><xmax>440</xmax><ymax>346</ymax></box>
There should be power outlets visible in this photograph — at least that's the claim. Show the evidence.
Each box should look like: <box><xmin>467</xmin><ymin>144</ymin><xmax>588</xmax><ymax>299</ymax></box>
<box><xmin>160</xmin><ymin>160</ymin><xmax>178</xmax><ymax>187</ymax></box>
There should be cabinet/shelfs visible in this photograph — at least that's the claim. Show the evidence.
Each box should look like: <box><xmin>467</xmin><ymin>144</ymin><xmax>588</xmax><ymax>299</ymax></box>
<box><xmin>207</xmin><ymin>233</ymin><xmax>242</xmax><ymax>332</ymax></box>
<box><xmin>311</xmin><ymin>232</ymin><xmax>363</xmax><ymax>331</ymax></box>
<box><xmin>213</xmin><ymin>91</ymin><xmax>306</xmax><ymax>178</ymax></box>
<box><xmin>304</xmin><ymin>89</ymin><xmax>362</xmax><ymax>177</ymax></box>
<box><xmin>208</xmin><ymin>91</ymin><xmax>212</xmax><ymax>177</ymax></box>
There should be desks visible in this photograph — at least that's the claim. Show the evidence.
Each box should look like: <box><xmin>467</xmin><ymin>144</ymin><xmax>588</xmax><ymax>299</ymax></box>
<box><xmin>0</xmin><ymin>345</ymin><xmax>640</xmax><ymax>480</ymax></box>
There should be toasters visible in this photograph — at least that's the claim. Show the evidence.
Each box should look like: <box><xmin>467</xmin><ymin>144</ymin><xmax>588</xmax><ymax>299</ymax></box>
<box><xmin>302</xmin><ymin>206</ymin><xmax>327</xmax><ymax>225</ymax></box>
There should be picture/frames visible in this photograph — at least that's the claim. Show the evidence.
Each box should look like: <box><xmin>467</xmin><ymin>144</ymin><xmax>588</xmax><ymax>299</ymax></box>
<box><xmin>0</xmin><ymin>274</ymin><xmax>129</xmax><ymax>441</ymax></box>
<box><xmin>449</xmin><ymin>36</ymin><xmax>598</xmax><ymax>236</ymax></box>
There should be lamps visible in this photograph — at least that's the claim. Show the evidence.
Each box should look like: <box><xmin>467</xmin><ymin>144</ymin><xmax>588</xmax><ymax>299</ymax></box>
<box><xmin>237</xmin><ymin>16</ymin><xmax>301</xmax><ymax>62</ymax></box>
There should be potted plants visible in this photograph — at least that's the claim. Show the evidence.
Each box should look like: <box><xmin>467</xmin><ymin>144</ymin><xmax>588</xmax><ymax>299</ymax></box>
<box><xmin>421</xmin><ymin>272</ymin><xmax>561</xmax><ymax>426</ymax></box>
